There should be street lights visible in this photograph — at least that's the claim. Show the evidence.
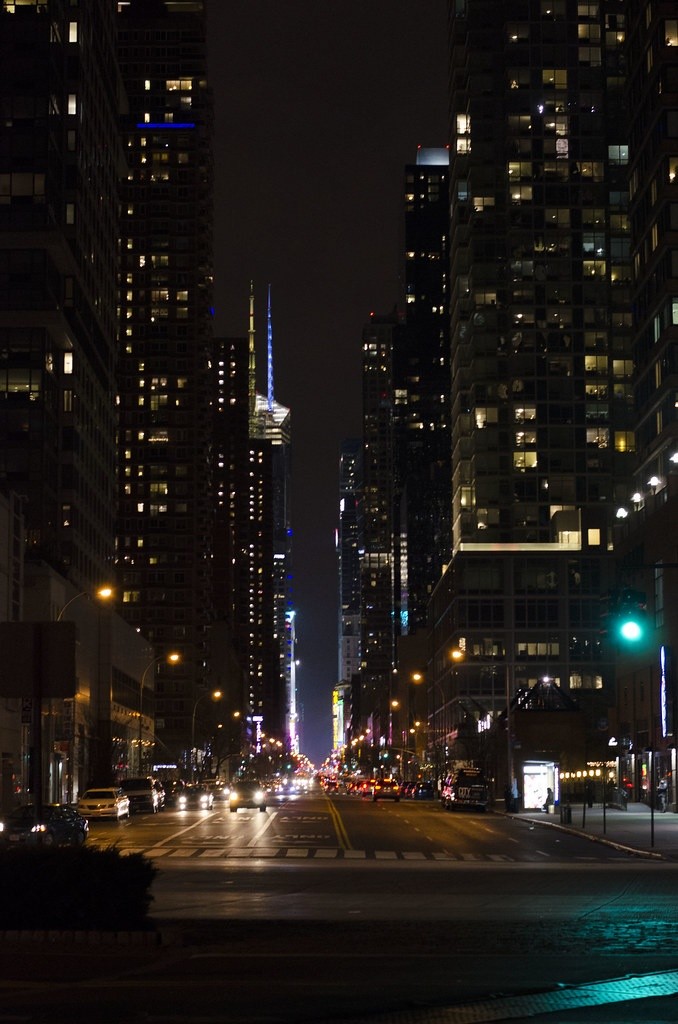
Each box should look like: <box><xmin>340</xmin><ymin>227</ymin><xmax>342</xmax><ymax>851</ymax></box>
<box><xmin>449</xmin><ymin>648</ymin><xmax>519</xmax><ymax>813</ymax></box>
<box><xmin>49</xmin><ymin>583</ymin><xmax>118</xmax><ymax>802</ymax></box>
<box><xmin>139</xmin><ymin>651</ymin><xmax>181</xmax><ymax>775</ymax></box>
<box><xmin>412</xmin><ymin>672</ymin><xmax>446</xmax><ymax>777</ymax></box>
<box><xmin>192</xmin><ymin>690</ymin><xmax>222</xmax><ymax>783</ymax></box>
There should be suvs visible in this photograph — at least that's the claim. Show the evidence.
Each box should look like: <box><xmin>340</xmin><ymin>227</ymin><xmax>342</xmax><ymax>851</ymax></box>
<box><xmin>119</xmin><ymin>775</ymin><xmax>166</xmax><ymax>813</ymax></box>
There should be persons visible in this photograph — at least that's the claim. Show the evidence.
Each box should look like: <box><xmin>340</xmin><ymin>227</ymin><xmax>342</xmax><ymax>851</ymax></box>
<box><xmin>543</xmin><ymin>787</ymin><xmax>554</xmax><ymax>814</ymax></box>
<box><xmin>656</xmin><ymin>778</ymin><xmax>668</xmax><ymax>813</ymax></box>
<box><xmin>504</xmin><ymin>783</ymin><xmax>511</xmax><ymax>812</ymax></box>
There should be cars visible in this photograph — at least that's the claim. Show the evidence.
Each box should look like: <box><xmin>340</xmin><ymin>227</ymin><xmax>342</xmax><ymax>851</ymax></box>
<box><xmin>315</xmin><ymin>770</ymin><xmax>489</xmax><ymax>813</ymax></box>
<box><xmin>77</xmin><ymin>787</ymin><xmax>130</xmax><ymax>822</ymax></box>
<box><xmin>165</xmin><ymin>777</ymin><xmax>292</xmax><ymax>812</ymax></box>
<box><xmin>0</xmin><ymin>803</ymin><xmax>89</xmax><ymax>858</ymax></box>
